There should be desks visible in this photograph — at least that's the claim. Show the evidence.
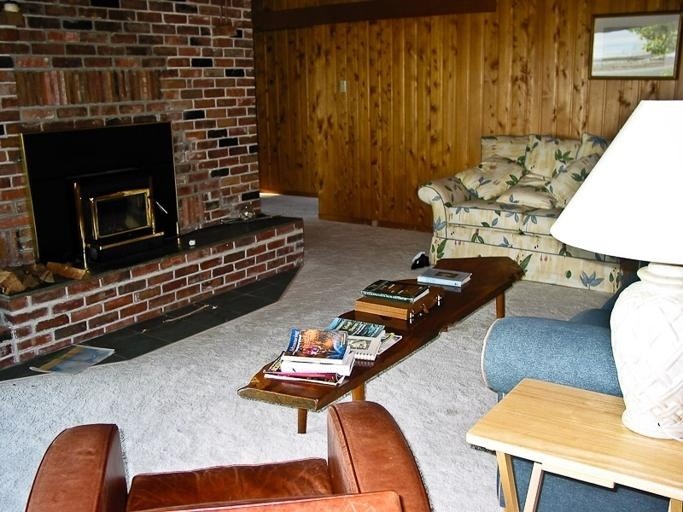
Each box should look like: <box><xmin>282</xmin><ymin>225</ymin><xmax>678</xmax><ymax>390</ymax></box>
<box><xmin>238</xmin><ymin>257</ymin><xmax>524</xmax><ymax>433</ymax></box>
<box><xmin>466</xmin><ymin>378</ymin><xmax>683</xmax><ymax>512</ymax></box>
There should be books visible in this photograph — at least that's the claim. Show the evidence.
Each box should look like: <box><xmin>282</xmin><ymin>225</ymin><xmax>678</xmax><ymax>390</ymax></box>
<box><xmin>261</xmin><ymin>263</ymin><xmax>472</xmax><ymax>389</ymax></box>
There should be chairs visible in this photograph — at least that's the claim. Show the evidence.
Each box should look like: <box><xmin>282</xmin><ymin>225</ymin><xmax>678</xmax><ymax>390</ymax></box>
<box><xmin>26</xmin><ymin>401</ymin><xmax>432</xmax><ymax>512</ymax></box>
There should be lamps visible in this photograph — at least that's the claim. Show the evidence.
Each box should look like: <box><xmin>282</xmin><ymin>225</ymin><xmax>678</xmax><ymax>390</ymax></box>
<box><xmin>550</xmin><ymin>99</ymin><xmax>683</xmax><ymax>440</ymax></box>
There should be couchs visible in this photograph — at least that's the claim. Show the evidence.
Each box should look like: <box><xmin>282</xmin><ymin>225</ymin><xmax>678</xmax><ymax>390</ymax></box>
<box><xmin>482</xmin><ymin>278</ymin><xmax>683</xmax><ymax>512</ymax></box>
<box><xmin>418</xmin><ymin>177</ymin><xmax>623</xmax><ymax>294</ymax></box>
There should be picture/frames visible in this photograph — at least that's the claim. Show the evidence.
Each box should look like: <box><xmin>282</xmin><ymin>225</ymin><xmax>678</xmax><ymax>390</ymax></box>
<box><xmin>589</xmin><ymin>11</ymin><xmax>683</xmax><ymax>79</ymax></box>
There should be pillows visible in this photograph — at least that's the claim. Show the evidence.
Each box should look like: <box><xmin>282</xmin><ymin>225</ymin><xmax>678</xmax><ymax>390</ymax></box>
<box><xmin>455</xmin><ymin>133</ymin><xmax>613</xmax><ymax>210</ymax></box>
